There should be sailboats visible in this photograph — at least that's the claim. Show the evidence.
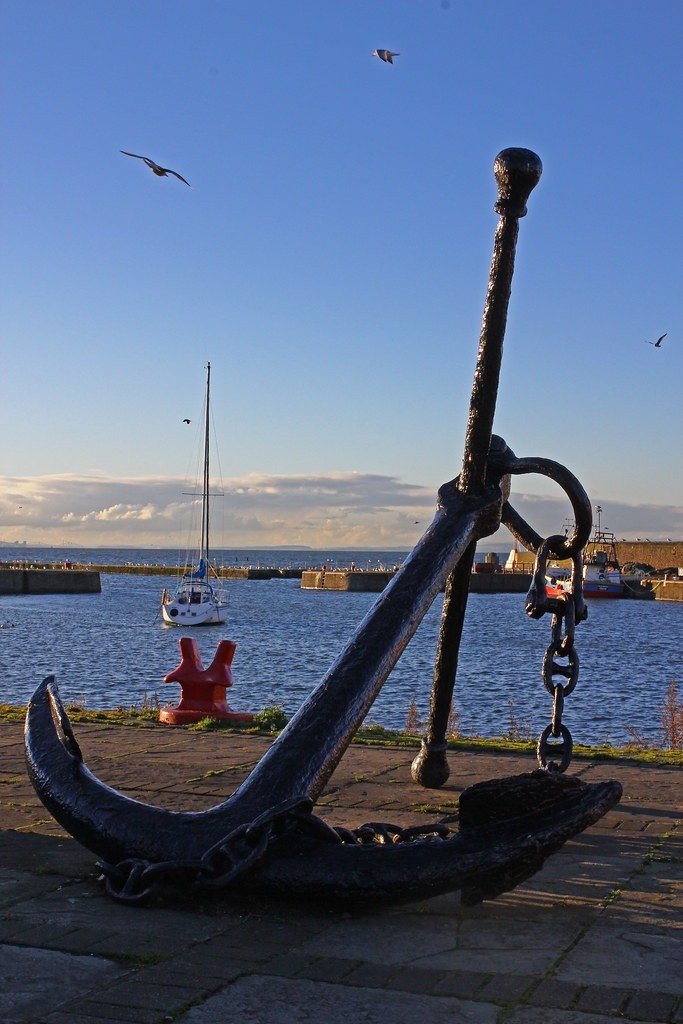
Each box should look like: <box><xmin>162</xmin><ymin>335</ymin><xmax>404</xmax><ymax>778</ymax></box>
<box><xmin>159</xmin><ymin>362</ymin><xmax>229</xmax><ymax>625</ymax></box>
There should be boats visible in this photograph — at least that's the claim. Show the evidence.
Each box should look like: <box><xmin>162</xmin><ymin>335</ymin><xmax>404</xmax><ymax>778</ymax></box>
<box><xmin>544</xmin><ymin>506</ymin><xmax>624</xmax><ymax>598</ymax></box>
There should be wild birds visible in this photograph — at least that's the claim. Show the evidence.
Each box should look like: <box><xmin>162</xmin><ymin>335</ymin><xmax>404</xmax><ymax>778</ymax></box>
<box><xmin>183</xmin><ymin>419</ymin><xmax>190</xmax><ymax>424</ymax></box>
<box><xmin>649</xmin><ymin>333</ymin><xmax>667</xmax><ymax>347</ymax></box>
<box><xmin>120</xmin><ymin>151</ymin><xmax>190</xmax><ymax>186</ymax></box>
<box><xmin>372</xmin><ymin>49</ymin><xmax>400</xmax><ymax>65</ymax></box>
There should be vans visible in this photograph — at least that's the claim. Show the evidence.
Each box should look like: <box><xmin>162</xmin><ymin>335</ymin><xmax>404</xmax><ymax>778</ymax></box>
<box><xmin>475</xmin><ymin>563</ymin><xmax>502</xmax><ymax>574</ymax></box>
